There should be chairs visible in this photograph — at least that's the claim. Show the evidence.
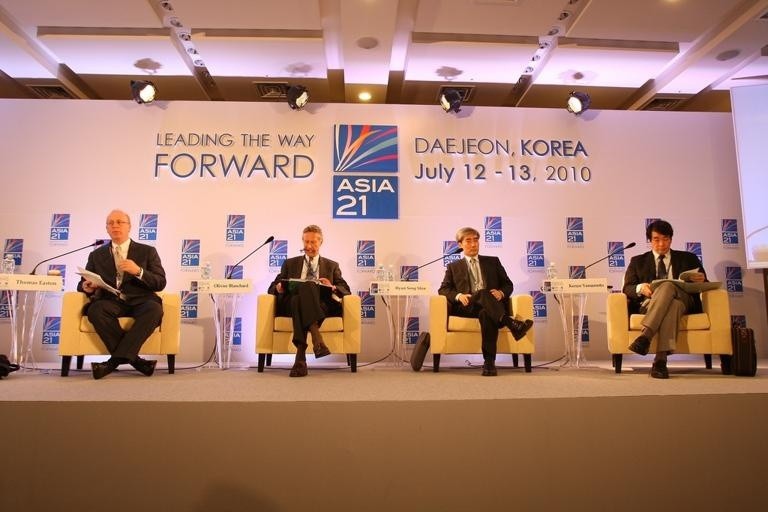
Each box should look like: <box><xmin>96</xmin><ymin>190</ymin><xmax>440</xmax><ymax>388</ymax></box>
<box><xmin>59</xmin><ymin>292</ymin><xmax>181</xmax><ymax>377</ymax></box>
<box><xmin>606</xmin><ymin>288</ymin><xmax>733</xmax><ymax>373</ymax></box>
<box><xmin>429</xmin><ymin>295</ymin><xmax>535</xmax><ymax>372</ymax></box>
<box><xmin>255</xmin><ymin>293</ymin><xmax>361</xmax><ymax>372</ymax></box>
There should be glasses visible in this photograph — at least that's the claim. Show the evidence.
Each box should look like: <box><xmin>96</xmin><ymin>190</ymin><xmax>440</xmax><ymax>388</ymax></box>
<box><xmin>106</xmin><ymin>220</ymin><xmax>129</xmax><ymax>224</ymax></box>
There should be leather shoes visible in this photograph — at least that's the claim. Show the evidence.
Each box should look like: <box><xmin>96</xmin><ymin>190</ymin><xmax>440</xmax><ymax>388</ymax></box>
<box><xmin>312</xmin><ymin>342</ymin><xmax>331</xmax><ymax>359</ymax></box>
<box><xmin>482</xmin><ymin>358</ymin><xmax>498</xmax><ymax>376</ymax></box>
<box><xmin>289</xmin><ymin>360</ymin><xmax>308</xmax><ymax>377</ymax></box>
<box><xmin>629</xmin><ymin>336</ymin><xmax>651</xmax><ymax>356</ymax></box>
<box><xmin>651</xmin><ymin>360</ymin><xmax>670</xmax><ymax>379</ymax></box>
<box><xmin>510</xmin><ymin>318</ymin><xmax>533</xmax><ymax>341</ymax></box>
<box><xmin>91</xmin><ymin>361</ymin><xmax>112</xmax><ymax>379</ymax></box>
<box><xmin>1</xmin><ymin>363</ymin><xmax>20</xmax><ymax>373</ymax></box>
<box><xmin>141</xmin><ymin>358</ymin><xmax>157</xmax><ymax>377</ymax></box>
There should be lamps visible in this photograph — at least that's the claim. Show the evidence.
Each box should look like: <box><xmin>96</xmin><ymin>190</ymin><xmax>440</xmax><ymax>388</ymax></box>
<box><xmin>282</xmin><ymin>84</ymin><xmax>309</xmax><ymax>111</ymax></box>
<box><xmin>129</xmin><ymin>80</ymin><xmax>158</xmax><ymax>104</ymax></box>
<box><xmin>566</xmin><ymin>90</ymin><xmax>591</xmax><ymax>114</ymax></box>
<box><xmin>439</xmin><ymin>90</ymin><xmax>463</xmax><ymax>113</ymax></box>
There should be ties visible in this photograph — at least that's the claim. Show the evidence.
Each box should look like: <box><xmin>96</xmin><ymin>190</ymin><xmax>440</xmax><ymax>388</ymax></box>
<box><xmin>115</xmin><ymin>248</ymin><xmax>124</xmax><ymax>277</ymax></box>
<box><xmin>468</xmin><ymin>258</ymin><xmax>480</xmax><ymax>291</ymax></box>
<box><xmin>657</xmin><ymin>254</ymin><xmax>668</xmax><ymax>278</ymax></box>
<box><xmin>306</xmin><ymin>256</ymin><xmax>316</xmax><ymax>281</ymax></box>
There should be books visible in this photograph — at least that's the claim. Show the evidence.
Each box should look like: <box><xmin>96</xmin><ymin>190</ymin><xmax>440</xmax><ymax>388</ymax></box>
<box><xmin>648</xmin><ymin>267</ymin><xmax>722</xmax><ymax>294</ymax></box>
<box><xmin>281</xmin><ymin>277</ymin><xmax>332</xmax><ymax>295</ymax></box>
<box><xmin>76</xmin><ymin>266</ymin><xmax>121</xmax><ymax>297</ymax></box>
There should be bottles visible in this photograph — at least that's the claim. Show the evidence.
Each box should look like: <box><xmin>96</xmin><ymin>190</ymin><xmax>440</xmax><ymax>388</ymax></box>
<box><xmin>375</xmin><ymin>263</ymin><xmax>396</xmax><ymax>281</ymax></box>
<box><xmin>200</xmin><ymin>260</ymin><xmax>214</xmax><ymax>281</ymax></box>
<box><xmin>546</xmin><ymin>261</ymin><xmax>559</xmax><ymax>281</ymax></box>
<box><xmin>2</xmin><ymin>253</ymin><xmax>16</xmax><ymax>276</ymax></box>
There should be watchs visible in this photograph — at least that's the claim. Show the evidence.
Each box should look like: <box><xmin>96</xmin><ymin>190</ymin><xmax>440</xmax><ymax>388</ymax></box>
<box><xmin>135</xmin><ymin>266</ymin><xmax>143</xmax><ymax>277</ymax></box>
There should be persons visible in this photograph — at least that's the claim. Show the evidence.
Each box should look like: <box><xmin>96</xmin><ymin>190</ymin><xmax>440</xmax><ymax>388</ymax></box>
<box><xmin>77</xmin><ymin>210</ymin><xmax>167</xmax><ymax>380</ymax></box>
<box><xmin>267</xmin><ymin>224</ymin><xmax>351</xmax><ymax>377</ymax></box>
<box><xmin>622</xmin><ymin>220</ymin><xmax>711</xmax><ymax>378</ymax></box>
<box><xmin>438</xmin><ymin>227</ymin><xmax>533</xmax><ymax>377</ymax></box>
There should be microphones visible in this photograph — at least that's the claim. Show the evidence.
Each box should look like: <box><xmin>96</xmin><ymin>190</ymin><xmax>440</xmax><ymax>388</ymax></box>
<box><xmin>572</xmin><ymin>241</ymin><xmax>636</xmax><ymax>279</ymax></box>
<box><xmin>226</xmin><ymin>236</ymin><xmax>275</xmax><ymax>279</ymax></box>
<box><xmin>30</xmin><ymin>241</ymin><xmax>104</xmax><ymax>275</ymax></box>
<box><xmin>402</xmin><ymin>248</ymin><xmax>463</xmax><ymax>282</ymax></box>
